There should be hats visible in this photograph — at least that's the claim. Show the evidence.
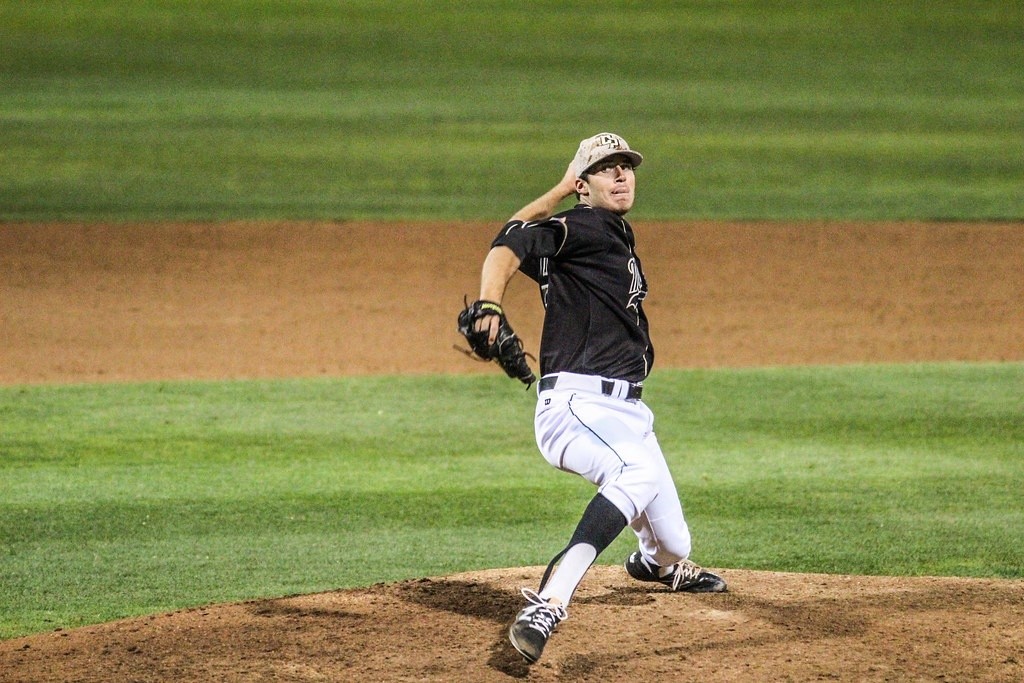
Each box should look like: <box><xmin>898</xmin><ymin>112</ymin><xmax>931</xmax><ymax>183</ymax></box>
<box><xmin>576</xmin><ymin>132</ymin><xmax>643</xmax><ymax>180</ymax></box>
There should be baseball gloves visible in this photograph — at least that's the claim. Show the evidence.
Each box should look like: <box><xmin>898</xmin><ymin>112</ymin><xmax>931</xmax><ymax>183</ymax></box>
<box><xmin>451</xmin><ymin>292</ymin><xmax>538</xmax><ymax>392</ymax></box>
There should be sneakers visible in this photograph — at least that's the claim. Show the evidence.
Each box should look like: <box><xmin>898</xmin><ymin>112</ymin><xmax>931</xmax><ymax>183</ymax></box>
<box><xmin>510</xmin><ymin>587</ymin><xmax>568</xmax><ymax>664</ymax></box>
<box><xmin>626</xmin><ymin>552</ymin><xmax>728</xmax><ymax>591</ymax></box>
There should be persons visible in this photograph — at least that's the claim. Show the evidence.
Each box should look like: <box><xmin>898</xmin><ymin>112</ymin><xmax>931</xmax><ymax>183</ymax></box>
<box><xmin>459</xmin><ymin>133</ymin><xmax>726</xmax><ymax>665</ymax></box>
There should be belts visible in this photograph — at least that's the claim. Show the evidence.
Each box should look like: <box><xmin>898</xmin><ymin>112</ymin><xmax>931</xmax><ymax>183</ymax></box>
<box><xmin>539</xmin><ymin>377</ymin><xmax>643</xmax><ymax>400</ymax></box>
